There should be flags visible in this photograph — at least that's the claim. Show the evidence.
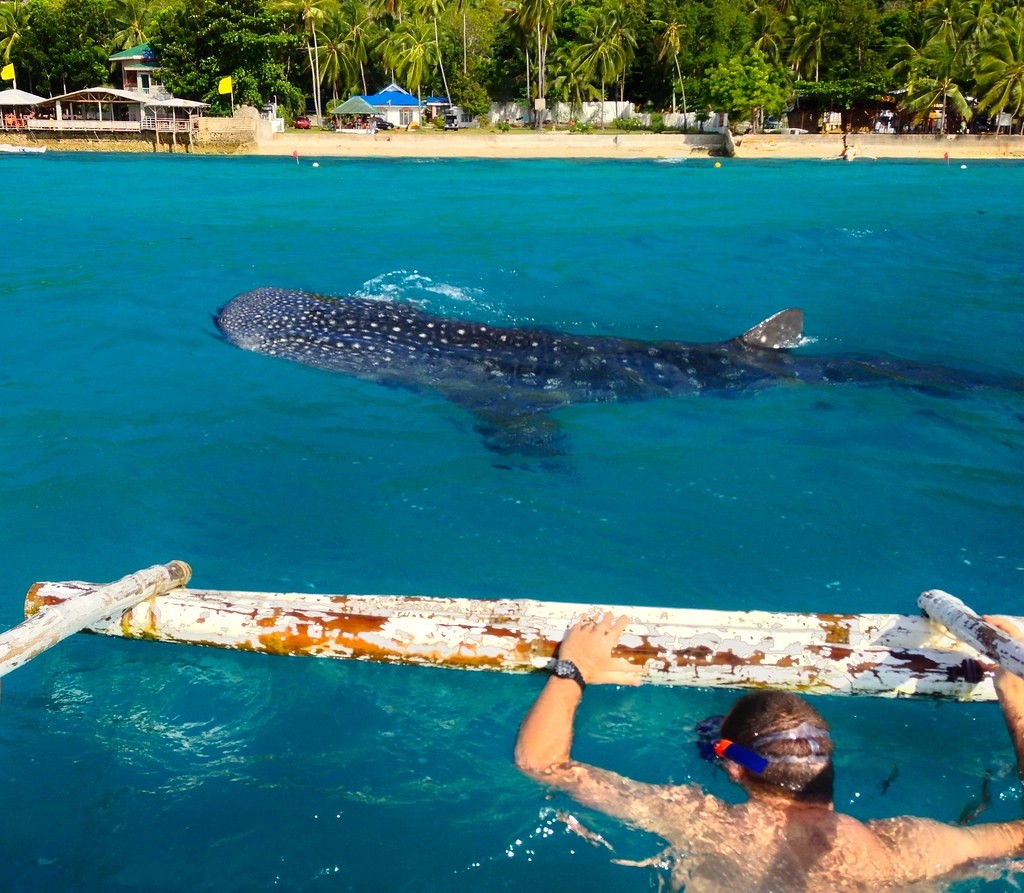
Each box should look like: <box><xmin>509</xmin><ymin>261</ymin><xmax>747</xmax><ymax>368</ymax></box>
<box><xmin>1</xmin><ymin>63</ymin><xmax>14</xmax><ymax>80</ymax></box>
<box><xmin>218</xmin><ymin>76</ymin><xmax>232</xmax><ymax>94</ymax></box>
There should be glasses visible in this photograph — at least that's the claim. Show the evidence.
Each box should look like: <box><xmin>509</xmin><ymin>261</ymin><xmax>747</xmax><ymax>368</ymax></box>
<box><xmin>694</xmin><ymin>714</ymin><xmax>835</xmax><ymax>771</ymax></box>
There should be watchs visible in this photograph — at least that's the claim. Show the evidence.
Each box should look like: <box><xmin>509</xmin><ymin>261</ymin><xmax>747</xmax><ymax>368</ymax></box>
<box><xmin>552</xmin><ymin>660</ymin><xmax>584</xmax><ymax>689</ymax></box>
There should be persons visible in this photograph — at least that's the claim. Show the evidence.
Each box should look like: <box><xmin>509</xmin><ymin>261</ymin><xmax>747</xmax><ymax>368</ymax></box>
<box><xmin>512</xmin><ymin>613</ymin><xmax>1024</xmax><ymax>893</ymax></box>
<box><xmin>883</xmin><ymin>118</ymin><xmax>970</xmax><ymax>134</ymax></box>
<box><xmin>326</xmin><ymin>108</ymin><xmax>511</xmax><ymax>130</ymax></box>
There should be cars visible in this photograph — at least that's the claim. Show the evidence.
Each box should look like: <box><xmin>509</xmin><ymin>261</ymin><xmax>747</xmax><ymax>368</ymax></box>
<box><xmin>444</xmin><ymin>113</ymin><xmax>459</xmax><ymax>132</ymax></box>
<box><xmin>375</xmin><ymin>117</ymin><xmax>395</xmax><ymax>130</ymax></box>
<box><xmin>295</xmin><ymin>116</ymin><xmax>311</xmax><ymax>128</ymax></box>
